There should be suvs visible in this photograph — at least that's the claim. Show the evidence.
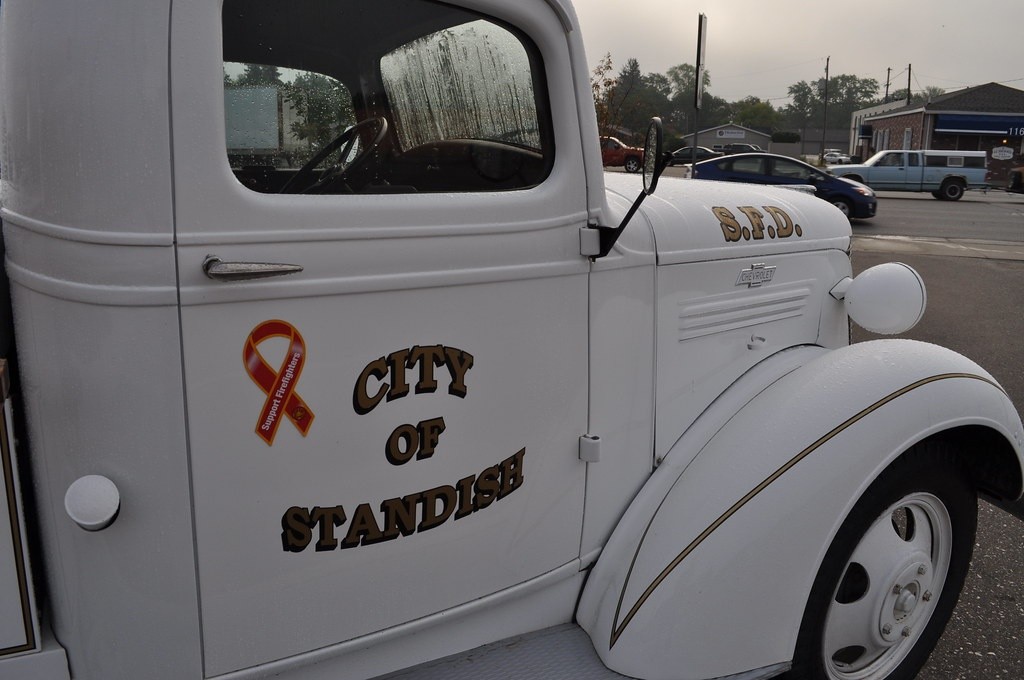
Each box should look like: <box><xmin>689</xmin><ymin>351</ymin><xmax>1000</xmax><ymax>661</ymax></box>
<box><xmin>725</xmin><ymin>142</ymin><xmax>767</xmax><ymax>154</ymax></box>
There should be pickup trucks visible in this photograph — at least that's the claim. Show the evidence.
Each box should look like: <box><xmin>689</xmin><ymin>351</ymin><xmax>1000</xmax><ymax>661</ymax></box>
<box><xmin>823</xmin><ymin>149</ymin><xmax>992</xmax><ymax>202</ymax></box>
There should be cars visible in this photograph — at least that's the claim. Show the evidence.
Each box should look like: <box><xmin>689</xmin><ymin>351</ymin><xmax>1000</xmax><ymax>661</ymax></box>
<box><xmin>1006</xmin><ymin>166</ymin><xmax>1024</xmax><ymax>192</ymax></box>
<box><xmin>683</xmin><ymin>152</ymin><xmax>877</xmax><ymax>223</ymax></box>
<box><xmin>823</xmin><ymin>152</ymin><xmax>851</xmax><ymax>164</ymax></box>
<box><xmin>2</xmin><ymin>1</ymin><xmax>1024</xmax><ymax>679</ymax></box>
<box><xmin>664</xmin><ymin>146</ymin><xmax>725</xmax><ymax>168</ymax></box>
<box><xmin>600</xmin><ymin>135</ymin><xmax>646</xmax><ymax>173</ymax></box>
